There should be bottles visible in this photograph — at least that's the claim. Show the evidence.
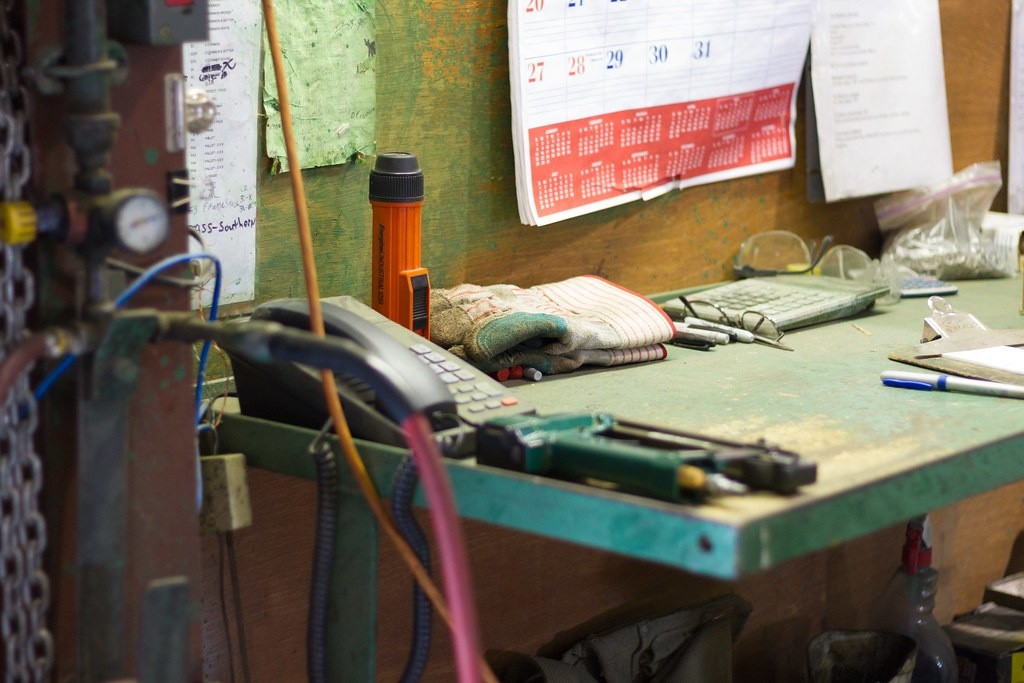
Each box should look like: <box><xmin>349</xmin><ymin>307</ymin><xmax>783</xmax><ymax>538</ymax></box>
<box><xmin>867</xmin><ymin>565</ymin><xmax>960</xmax><ymax>683</ymax></box>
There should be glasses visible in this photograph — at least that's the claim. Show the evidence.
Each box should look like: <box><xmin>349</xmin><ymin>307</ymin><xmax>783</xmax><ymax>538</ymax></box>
<box><xmin>679</xmin><ymin>296</ymin><xmax>785</xmax><ymax>343</ymax></box>
<box><xmin>733</xmin><ymin>230</ymin><xmax>900</xmax><ymax>307</ymax></box>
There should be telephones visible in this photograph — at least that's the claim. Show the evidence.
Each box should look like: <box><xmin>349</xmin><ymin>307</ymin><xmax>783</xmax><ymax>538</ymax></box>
<box><xmin>215</xmin><ymin>294</ymin><xmax>538</xmax><ymax>459</ymax></box>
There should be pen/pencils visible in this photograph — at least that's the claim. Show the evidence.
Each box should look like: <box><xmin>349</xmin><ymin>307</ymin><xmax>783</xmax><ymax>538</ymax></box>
<box><xmin>880</xmin><ymin>370</ymin><xmax>1024</xmax><ymax>400</ymax></box>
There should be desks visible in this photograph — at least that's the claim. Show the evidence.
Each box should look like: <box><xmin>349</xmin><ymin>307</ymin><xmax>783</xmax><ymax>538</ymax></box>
<box><xmin>200</xmin><ymin>254</ymin><xmax>1024</xmax><ymax>683</ymax></box>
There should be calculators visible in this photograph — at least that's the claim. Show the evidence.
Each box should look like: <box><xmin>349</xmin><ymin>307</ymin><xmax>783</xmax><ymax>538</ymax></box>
<box><xmin>845</xmin><ymin>263</ymin><xmax>957</xmax><ymax>299</ymax></box>
<box><xmin>664</xmin><ymin>272</ymin><xmax>890</xmax><ymax>333</ymax></box>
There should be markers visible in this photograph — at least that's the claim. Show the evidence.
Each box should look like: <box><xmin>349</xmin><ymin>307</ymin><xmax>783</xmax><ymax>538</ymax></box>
<box><xmin>663</xmin><ymin>314</ymin><xmax>755</xmax><ymax>352</ymax></box>
